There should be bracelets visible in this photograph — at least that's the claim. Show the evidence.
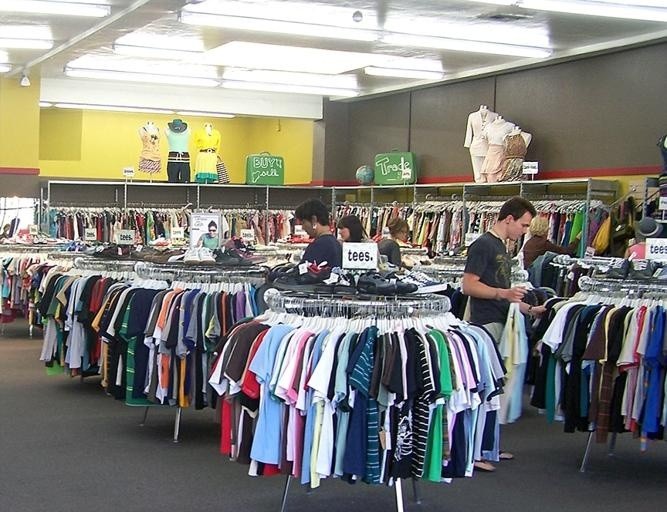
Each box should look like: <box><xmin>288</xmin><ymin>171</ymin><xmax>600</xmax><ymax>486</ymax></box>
<box><xmin>528</xmin><ymin>305</ymin><xmax>533</xmax><ymax>315</ymax></box>
<box><xmin>494</xmin><ymin>287</ymin><xmax>500</xmax><ymax>302</ymax></box>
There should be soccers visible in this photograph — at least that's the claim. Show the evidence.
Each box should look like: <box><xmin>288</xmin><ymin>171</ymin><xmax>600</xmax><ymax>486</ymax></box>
<box><xmin>356</xmin><ymin>166</ymin><xmax>375</xmax><ymax>184</ymax></box>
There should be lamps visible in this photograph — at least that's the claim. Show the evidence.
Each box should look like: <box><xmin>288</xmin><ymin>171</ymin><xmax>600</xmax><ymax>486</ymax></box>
<box><xmin>0</xmin><ymin>0</ymin><xmax>562</xmax><ymax>119</ymax></box>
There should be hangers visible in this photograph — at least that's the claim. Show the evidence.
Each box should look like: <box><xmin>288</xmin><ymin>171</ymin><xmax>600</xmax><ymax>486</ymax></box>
<box><xmin>396</xmin><ymin>245</ymin><xmax>666</xmax><ymax>320</ymax></box>
<box><xmin>47</xmin><ymin>202</ymin><xmax>304</xmax><ymax>228</ymax></box>
<box><xmin>336</xmin><ymin>193</ymin><xmax>663</xmax><ymax>219</ymax></box>
<box><xmin>2</xmin><ymin>236</ymin><xmax>311</xmax><ymax>296</ymax></box>
<box><xmin>248</xmin><ymin>291</ymin><xmax>471</xmax><ymax>339</ymax></box>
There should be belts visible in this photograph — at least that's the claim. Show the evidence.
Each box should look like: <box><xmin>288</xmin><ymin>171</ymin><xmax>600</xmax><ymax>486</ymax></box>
<box><xmin>200</xmin><ymin>149</ymin><xmax>215</xmax><ymax>153</ymax></box>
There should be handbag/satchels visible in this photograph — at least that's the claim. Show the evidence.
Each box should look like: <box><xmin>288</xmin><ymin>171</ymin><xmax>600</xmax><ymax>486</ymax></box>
<box><xmin>591</xmin><ymin>214</ymin><xmax>611</xmax><ymax>254</ymax></box>
<box><xmin>613</xmin><ymin>221</ymin><xmax>632</xmax><ymax>240</ymax></box>
<box><xmin>216</xmin><ymin>158</ymin><xmax>230</xmax><ymax>183</ymax></box>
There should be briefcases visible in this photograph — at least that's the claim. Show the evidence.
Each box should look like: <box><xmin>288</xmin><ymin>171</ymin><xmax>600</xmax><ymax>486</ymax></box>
<box><xmin>246</xmin><ymin>152</ymin><xmax>284</xmax><ymax>185</ymax></box>
<box><xmin>374</xmin><ymin>148</ymin><xmax>417</xmax><ymax>186</ymax></box>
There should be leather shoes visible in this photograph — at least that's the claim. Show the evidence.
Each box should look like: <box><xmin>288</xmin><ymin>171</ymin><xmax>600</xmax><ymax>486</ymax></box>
<box><xmin>60</xmin><ymin>240</ymin><xmax>87</xmax><ymax>251</ymax></box>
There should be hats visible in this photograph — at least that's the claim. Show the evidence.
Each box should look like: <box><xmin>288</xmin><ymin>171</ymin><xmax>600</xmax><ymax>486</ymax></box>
<box><xmin>168</xmin><ymin>119</ymin><xmax>187</xmax><ymax>133</ymax></box>
<box><xmin>634</xmin><ymin>218</ymin><xmax>663</xmax><ymax>241</ymax></box>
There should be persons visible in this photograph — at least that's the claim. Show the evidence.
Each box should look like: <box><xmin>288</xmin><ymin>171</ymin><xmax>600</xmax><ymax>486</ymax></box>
<box><xmin>463</xmin><ymin>194</ymin><xmax>547</xmax><ymax>471</ymax></box>
<box><xmin>196</xmin><ymin>122</ymin><xmax>221</xmax><ymax>183</ymax></box>
<box><xmin>625</xmin><ymin>217</ymin><xmax>664</xmax><ymax>259</ymax></box>
<box><xmin>337</xmin><ymin>216</ymin><xmax>380</xmax><ymax>259</ymax></box>
<box><xmin>497</xmin><ymin>126</ymin><xmax>532</xmax><ymax>182</ymax></box>
<box><xmin>139</xmin><ymin>122</ymin><xmax>161</xmax><ymax>174</ymax></box>
<box><xmin>523</xmin><ymin>216</ymin><xmax>581</xmax><ymax>271</ymax></box>
<box><xmin>482</xmin><ymin>116</ymin><xmax>516</xmax><ymax>183</ymax></box>
<box><xmin>165</xmin><ymin>119</ymin><xmax>190</xmax><ymax>182</ymax></box>
<box><xmin>197</xmin><ymin>220</ymin><xmax>218</xmax><ymax>249</ymax></box>
<box><xmin>464</xmin><ymin>105</ymin><xmax>498</xmax><ymax>182</ymax></box>
<box><xmin>296</xmin><ymin>199</ymin><xmax>342</xmax><ymax>267</ymax></box>
<box><xmin>380</xmin><ymin>217</ymin><xmax>410</xmax><ymax>268</ymax></box>
<box><xmin>2</xmin><ymin>224</ymin><xmax>11</xmax><ymax>238</ymax></box>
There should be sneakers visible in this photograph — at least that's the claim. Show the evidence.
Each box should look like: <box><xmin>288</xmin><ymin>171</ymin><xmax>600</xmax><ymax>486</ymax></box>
<box><xmin>474</xmin><ymin>459</ymin><xmax>495</xmax><ymax>471</ymax></box>
<box><xmin>0</xmin><ymin>232</ymin><xmax>57</xmax><ymax>245</ymax></box>
<box><xmin>595</xmin><ymin>257</ymin><xmax>667</xmax><ymax>281</ymax></box>
<box><xmin>268</xmin><ymin>262</ymin><xmax>448</xmax><ymax>294</ymax></box>
<box><xmin>93</xmin><ymin>237</ymin><xmax>267</xmax><ymax>266</ymax></box>
<box><xmin>499</xmin><ymin>452</ymin><xmax>514</xmax><ymax>459</ymax></box>
